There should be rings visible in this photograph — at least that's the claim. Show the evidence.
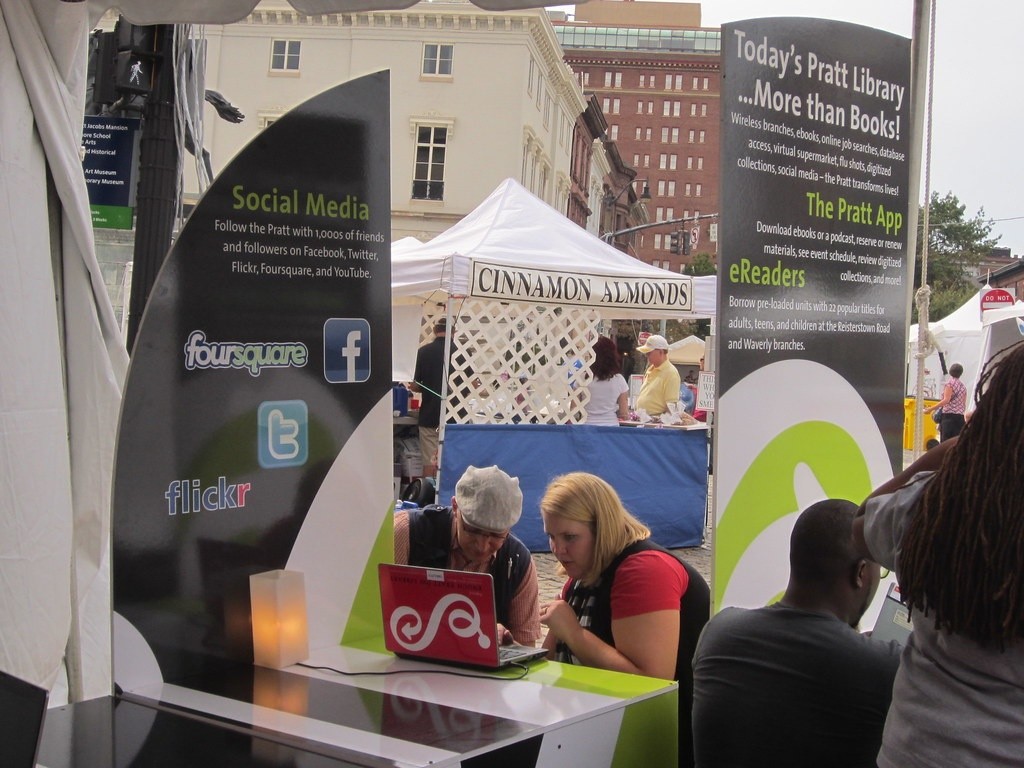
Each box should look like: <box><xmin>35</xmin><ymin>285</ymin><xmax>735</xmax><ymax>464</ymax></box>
<box><xmin>545</xmin><ymin>608</ymin><xmax>547</xmax><ymax>613</ymax></box>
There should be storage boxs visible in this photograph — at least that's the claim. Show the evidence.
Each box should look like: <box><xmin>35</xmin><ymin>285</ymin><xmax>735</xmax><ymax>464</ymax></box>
<box><xmin>401</xmin><ymin>458</ymin><xmax>422</xmax><ymax>478</ymax></box>
<box><xmin>393</xmin><ymin>477</ymin><xmax>401</xmax><ymax>502</ymax></box>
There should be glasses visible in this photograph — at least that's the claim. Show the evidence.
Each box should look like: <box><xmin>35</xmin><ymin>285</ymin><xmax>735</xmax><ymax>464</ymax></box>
<box><xmin>460</xmin><ymin>511</ymin><xmax>508</xmax><ymax>542</ymax></box>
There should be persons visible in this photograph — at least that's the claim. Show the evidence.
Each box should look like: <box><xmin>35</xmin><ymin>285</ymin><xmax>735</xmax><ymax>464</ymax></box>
<box><xmin>567</xmin><ymin>335</ymin><xmax>629</xmax><ymax>426</ymax></box>
<box><xmin>851</xmin><ymin>340</ymin><xmax>1024</xmax><ymax>768</ymax></box>
<box><xmin>408</xmin><ymin>316</ymin><xmax>479</xmax><ymax>477</ymax></box>
<box><xmin>691</xmin><ymin>497</ymin><xmax>896</xmax><ymax>768</ymax></box>
<box><xmin>922</xmin><ymin>364</ymin><xmax>967</xmax><ymax>443</ymax></box>
<box><xmin>538</xmin><ymin>472</ymin><xmax>710</xmax><ymax>768</ymax></box>
<box><xmin>636</xmin><ymin>335</ymin><xmax>681</xmax><ymax>416</ymax></box>
<box><xmin>393</xmin><ymin>465</ymin><xmax>542</xmax><ymax>648</ymax></box>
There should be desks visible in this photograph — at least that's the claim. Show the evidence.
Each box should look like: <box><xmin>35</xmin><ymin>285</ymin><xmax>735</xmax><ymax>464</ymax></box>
<box><xmin>616</xmin><ymin>418</ymin><xmax>706</xmax><ymax>430</ymax></box>
<box><xmin>392</xmin><ymin>415</ymin><xmax>419</xmax><ymax>427</ymax></box>
<box><xmin>117</xmin><ymin>654</ymin><xmax>682</xmax><ymax>768</ymax></box>
<box><xmin>33</xmin><ymin>687</ymin><xmax>386</xmax><ymax>768</ymax></box>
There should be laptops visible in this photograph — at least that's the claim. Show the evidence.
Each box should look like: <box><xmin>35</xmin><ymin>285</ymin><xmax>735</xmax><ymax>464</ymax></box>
<box><xmin>378</xmin><ymin>562</ymin><xmax>549</xmax><ymax>670</ymax></box>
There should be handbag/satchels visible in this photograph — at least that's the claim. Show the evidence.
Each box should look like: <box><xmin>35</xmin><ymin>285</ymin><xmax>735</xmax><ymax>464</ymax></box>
<box><xmin>932</xmin><ymin>407</ymin><xmax>942</xmax><ymax>423</ymax></box>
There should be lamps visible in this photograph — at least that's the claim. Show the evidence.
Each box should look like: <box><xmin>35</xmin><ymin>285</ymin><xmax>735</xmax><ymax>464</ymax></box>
<box><xmin>246</xmin><ymin>568</ymin><xmax>313</xmax><ymax>667</ymax></box>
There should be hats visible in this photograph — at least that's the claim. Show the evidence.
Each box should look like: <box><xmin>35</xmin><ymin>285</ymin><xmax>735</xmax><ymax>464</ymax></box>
<box><xmin>637</xmin><ymin>334</ymin><xmax>669</xmax><ymax>353</ymax></box>
<box><xmin>456</xmin><ymin>464</ymin><xmax>522</xmax><ymax>533</ymax></box>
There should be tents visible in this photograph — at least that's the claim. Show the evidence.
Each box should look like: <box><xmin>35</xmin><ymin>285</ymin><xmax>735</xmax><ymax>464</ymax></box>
<box><xmin>663</xmin><ymin>335</ymin><xmax>706</xmax><ymax>385</ymax></box>
<box><xmin>391</xmin><ymin>177</ymin><xmax>717</xmax><ymax>554</ymax></box>
<box><xmin>908</xmin><ymin>284</ymin><xmax>1024</xmax><ymax>421</ymax></box>
